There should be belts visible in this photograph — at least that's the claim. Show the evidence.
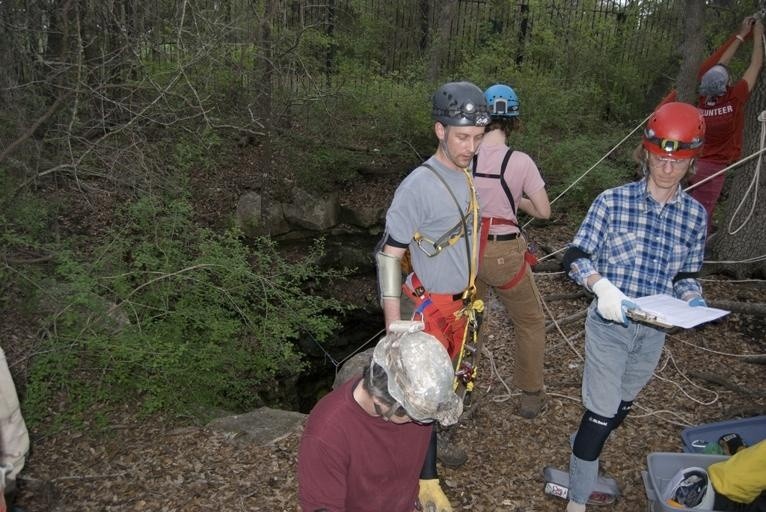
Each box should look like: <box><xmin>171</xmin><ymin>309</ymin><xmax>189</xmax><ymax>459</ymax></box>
<box><xmin>487</xmin><ymin>232</ymin><xmax>520</xmax><ymax>241</ymax></box>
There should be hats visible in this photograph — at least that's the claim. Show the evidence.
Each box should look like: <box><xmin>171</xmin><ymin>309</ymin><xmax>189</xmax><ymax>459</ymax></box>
<box><xmin>372</xmin><ymin>331</ymin><xmax>465</xmax><ymax>428</ymax></box>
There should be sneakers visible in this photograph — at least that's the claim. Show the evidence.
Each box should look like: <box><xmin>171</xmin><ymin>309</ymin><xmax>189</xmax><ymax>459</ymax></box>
<box><xmin>437</xmin><ymin>433</ymin><xmax>469</xmax><ymax>470</ymax></box>
<box><xmin>520</xmin><ymin>389</ymin><xmax>548</xmax><ymax>419</ymax></box>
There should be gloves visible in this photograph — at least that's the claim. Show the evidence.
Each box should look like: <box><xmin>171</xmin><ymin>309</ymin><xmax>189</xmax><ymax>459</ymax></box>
<box><xmin>418</xmin><ymin>479</ymin><xmax>452</xmax><ymax>512</ymax></box>
<box><xmin>592</xmin><ymin>277</ymin><xmax>639</xmax><ymax>326</ymax></box>
<box><xmin>687</xmin><ymin>297</ymin><xmax>708</xmax><ymax>307</ymax></box>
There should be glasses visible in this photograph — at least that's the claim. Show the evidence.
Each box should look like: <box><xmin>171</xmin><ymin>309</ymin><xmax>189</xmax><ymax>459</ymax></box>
<box><xmin>644</xmin><ymin>149</ymin><xmax>689</xmax><ymax>171</ymax></box>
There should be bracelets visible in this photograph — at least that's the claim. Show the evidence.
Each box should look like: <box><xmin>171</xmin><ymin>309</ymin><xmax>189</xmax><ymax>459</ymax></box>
<box><xmin>735</xmin><ymin>34</ymin><xmax>745</xmax><ymax>42</ymax></box>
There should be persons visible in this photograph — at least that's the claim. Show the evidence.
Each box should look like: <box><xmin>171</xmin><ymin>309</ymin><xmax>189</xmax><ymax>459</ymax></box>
<box><xmin>560</xmin><ymin>101</ymin><xmax>709</xmax><ymax>512</ymax></box>
<box><xmin>679</xmin><ymin>16</ymin><xmax>764</xmax><ymax>241</ymax></box>
<box><xmin>382</xmin><ymin>82</ymin><xmax>492</xmax><ymax>512</ymax></box>
<box><xmin>469</xmin><ymin>85</ymin><xmax>555</xmax><ymax>419</ymax></box>
<box><xmin>298</xmin><ymin>331</ymin><xmax>464</xmax><ymax>512</ymax></box>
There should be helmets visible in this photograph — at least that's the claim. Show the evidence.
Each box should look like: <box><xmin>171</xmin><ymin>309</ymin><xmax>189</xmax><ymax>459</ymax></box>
<box><xmin>484</xmin><ymin>84</ymin><xmax>520</xmax><ymax>117</ymax></box>
<box><xmin>641</xmin><ymin>102</ymin><xmax>706</xmax><ymax>159</ymax></box>
<box><xmin>431</xmin><ymin>81</ymin><xmax>488</xmax><ymax>126</ymax></box>
